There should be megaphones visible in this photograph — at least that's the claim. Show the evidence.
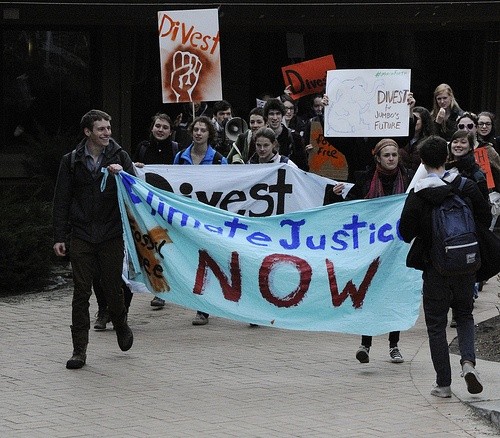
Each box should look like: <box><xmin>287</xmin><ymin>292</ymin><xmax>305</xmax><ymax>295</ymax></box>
<box><xmin>222</xmin><ymin>116</ymin><xmax>248</xmax><ymax>141</ymax></box>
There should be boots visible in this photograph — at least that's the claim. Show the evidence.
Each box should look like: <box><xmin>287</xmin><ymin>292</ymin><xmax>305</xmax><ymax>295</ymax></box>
<box><xmin>111</xmin><ymin>316</ymin><xmax>133</xmax><ymax>351</ymax></box>
<box><xmin>65</xmin><ymin>326</ymin><xmax>87</xmax><ymax>368</ymax></box>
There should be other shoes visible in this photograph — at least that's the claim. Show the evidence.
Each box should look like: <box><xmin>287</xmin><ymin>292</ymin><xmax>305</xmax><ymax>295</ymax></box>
<box><xmin>450</xmin><ymin>319</ymin><xmax>456</xmax><ymax>327</ymax></box>
<box><xmin>192</xmin><ymin>315</ymin><xmax>207</xmax><ymax>325</ymax></box>
<box><xmin>151</xmin><ymin>297</ymin><xmax>164</xmax><ymax>306</ymax></box>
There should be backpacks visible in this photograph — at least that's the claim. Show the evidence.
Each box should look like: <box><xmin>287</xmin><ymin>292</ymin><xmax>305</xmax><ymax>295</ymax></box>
<box><xmin>430</xmin><ymin>177</ymin><xmax>481</xmax><ymax>276</ymax></box>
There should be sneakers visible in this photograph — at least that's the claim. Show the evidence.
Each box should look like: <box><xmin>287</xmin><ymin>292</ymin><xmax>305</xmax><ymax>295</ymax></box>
<box><xmin>356</xmin><ymin>347</ymin><xmax>370</xmax><ymax>362</ymax></box>
<box><xmin>94</xmin><ymin>309</ymin><xmax>109</xmax><ymax>329</ymax></box>
<box><xmin>460</xmin><ymin>364</ymin><xmax>483</xmax><ymax>394</ymax></box>
<box><xmin>389</xmin><ymin>347</ymin><xmax>404</xmax><ymax>363</ymax></box>
<box><xmin>430</xmin><ymin>382</ymin><xmax>451</xmax><ymax>398</ymax></box>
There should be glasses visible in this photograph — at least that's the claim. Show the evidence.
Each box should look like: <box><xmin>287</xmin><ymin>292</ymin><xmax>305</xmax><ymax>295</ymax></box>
<box><xmin>458</xmin><ymin>123</ymin><xmax>475</xmax><ymax>129</ymax></box>
<box><xmin>478</xmin><ymin>122</ymin><xmax>492</xmax><ymax>128</ymax></box>
<box><xmin>286</xmin><ymin>107</ymin><xmax>294</xmax><ymax>112</ymax></box>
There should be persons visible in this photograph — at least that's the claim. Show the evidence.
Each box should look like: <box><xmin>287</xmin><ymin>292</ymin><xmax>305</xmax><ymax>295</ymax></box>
<box><xmin>324</xmin><ymin>139</ymin><xmax>410</xmax><ymax>363</ymax></box>
<box><xmin>399</xmin><ymin>136</ymin><xmax>493</xmax><ymax>397</ymax></box>
<box><xmin>50</xmin><ymin>109</ymin><xmax>137</xmax><ymax>370</ymax></box>
<box><xmin>51</xmin><ymin>83</ymin><xmax>500</xmax><ymax>330</ymax></box>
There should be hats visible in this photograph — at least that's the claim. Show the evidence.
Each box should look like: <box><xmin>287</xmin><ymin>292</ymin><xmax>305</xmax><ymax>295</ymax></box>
<box><xmin>374</xmin><ymin>138</ymin><xmax>398</xmax><ymax>155</ymax></box>
